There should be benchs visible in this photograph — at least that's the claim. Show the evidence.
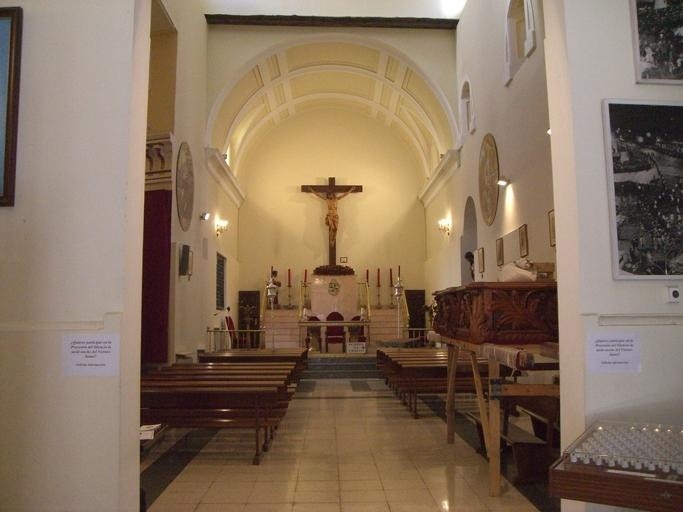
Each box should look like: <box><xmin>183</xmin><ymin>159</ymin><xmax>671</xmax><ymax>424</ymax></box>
<box><xmin>376</xmin><ymin>347</ymin><xmax>522</xmax><ymax>420</ymax></box>
<box><xmin>141</xmin><ymin>349</ymin><xmax>305</xmax><ymax>466</ymax></box>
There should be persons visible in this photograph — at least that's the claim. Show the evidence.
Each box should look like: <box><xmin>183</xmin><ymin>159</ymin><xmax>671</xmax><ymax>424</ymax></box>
<box><xmin>306</xmin><ymin>186</ymin><xmax>356</xmax><ymax>248</ymax></box>
<box><xmin>465</xmin><ymin>251</ymin><xmax>474</xmax><ymax>283</ymax></box>
<box><xmin>265</xmin><ymin>271</ymin><xmax>282</xmax><ymax>304</ymax></box>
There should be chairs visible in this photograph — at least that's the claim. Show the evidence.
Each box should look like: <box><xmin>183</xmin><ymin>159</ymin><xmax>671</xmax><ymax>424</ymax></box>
<box><xmin>304</xmin><ymin>311</ymin><xmax>364</xmax><ymax>352</ymax></box>
<box><xmin>226</xmin><ymin>316</ymin><xmax>256</xmax><ymax>349</ymax></box>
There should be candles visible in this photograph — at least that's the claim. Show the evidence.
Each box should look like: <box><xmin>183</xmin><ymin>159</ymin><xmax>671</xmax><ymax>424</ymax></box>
<box><xmin>287</xmin><ymin>269</ymin><xmax>291</xmax><ymax>287</ymax></box>
<box><xmin>378</xmin><ymin>268</ymin><xmax>380</xmax><ymax>286</ymax></box>
<box><xmin>366</xmin><ymin>269</ymin><xmax>369</xmax><ymax>281</ymax></box>
<box><xmin>389</xmin><ymin>265</ymin><xmax>400</xmax><ymax>284</ymax></box>
<box><xmin>304</xmin><ymin>269</ymin><xmax>307</xmax><ymax>282</ymax></box>
